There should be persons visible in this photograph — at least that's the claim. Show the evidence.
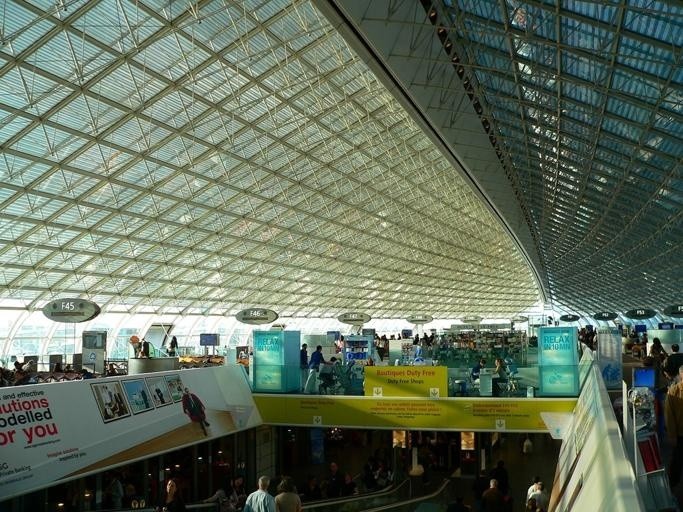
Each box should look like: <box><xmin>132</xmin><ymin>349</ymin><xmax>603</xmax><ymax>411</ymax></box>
<box><xmin>578</xmin><ymin>323</ymin><xmax>683</xmax><ymax>512</ymax></box>
<box><xmin>102</xmin><ymin>470</ymin><xmax>187</xmax><ymax>511</ymax></box>
<box><xmin>238</xmin><ymin>347</ymin><xmax>249</xmax><ymax>360</ymax></box>
<box><xmin>167</xmin><ymin>335</ymin><xmax>179</xmax><ymax>356</ymax></box>
<box><xmin>0</xmin><ymin>352</ymin><xmax>117</xmax><ymax>387</ymax></box>
<box><xmin>203</xmin><ymin>462</ymin><xmax>357</xmax><ymax>511</ymax></box>
<box><xmin>363</xmin><ymin>438</ymin><xmax>508</xmax><ymax>510</ymax></box>
<box><xmin>183</xmin><ymin>387</ymin><xmax>210</xmax><ymax>436</ymax></box>
<box><xmin>525</xmin><ymin>477</ymin><xmax>548</xmax><ymax>511</ymax></box>
<box><xmin>297</xmin><ymin>330</ymin><xmax>527</xmax><ymax>396</ymax></box>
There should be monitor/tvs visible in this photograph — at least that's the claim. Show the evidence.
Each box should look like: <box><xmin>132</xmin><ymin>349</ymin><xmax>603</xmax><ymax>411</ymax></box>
<box><xmin>83</xmin><ymin>332</ymin><xmax>105</xmax><ymax>349</ymax></box>
<box><xmin>635</xmin><ymin>325</ymin><xmax>646</xmax><ymax>332</ymax></box>
<box><xmin>402</xmin><ymin>329</ymin><xmax>412</xmax><ymax>336</ymax></box>
<box><xmin>328</xmin><ymin>331</ymin><xmax>340</xmax><ymax>340</ymax></box>
<box><xmin>632</xmin><ymin>367</ymin><xmax>658</xmax><ymax>388</ymax></box>
<box><xmin>200</xmin><ymin>334</ymin><xmax>217</xmax><ymax>346</ymax></box>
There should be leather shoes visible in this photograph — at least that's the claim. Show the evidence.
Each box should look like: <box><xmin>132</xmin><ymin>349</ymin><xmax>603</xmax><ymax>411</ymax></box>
<box><xmin>203</xmin><ymin>422</ymin><xmax>209</xmax><ymax>436</ymax></box>
